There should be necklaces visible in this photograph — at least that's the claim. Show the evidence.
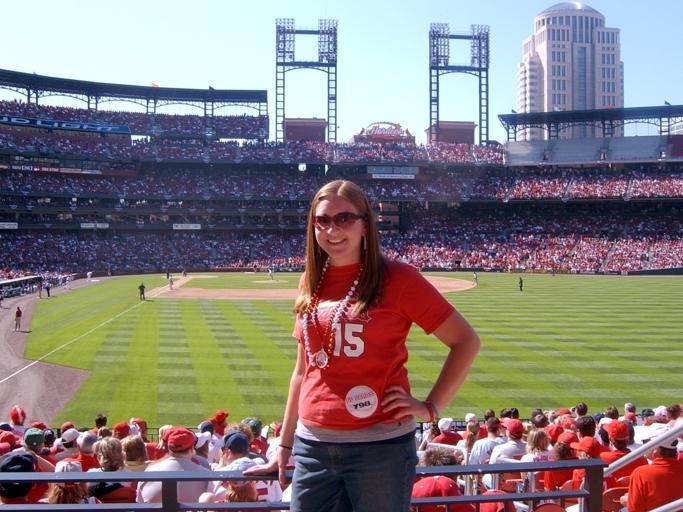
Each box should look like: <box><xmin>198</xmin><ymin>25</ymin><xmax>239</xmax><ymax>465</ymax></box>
<box><xmin>300</xmin><ymin>255</ymin><xmax>366</xmax><ymax>373</ymax></box>
<box><xmin>312</xmin><ymin>281</ymin><xmax>350</xmax><ymax>369</ymax></box>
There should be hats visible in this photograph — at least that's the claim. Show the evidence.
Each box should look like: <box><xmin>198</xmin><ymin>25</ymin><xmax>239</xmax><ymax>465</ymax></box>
<box><xmin>76</xmin><ymin>431</ymin><xmax>99</xmax><ymax>453</ymax></box>
<box><xmin>162</xmin><ymin>428</ymin><xmax>199</xmax><ymax>452</ymax></box>
<box><xmin>130</xmin><ymin>418</ymin><xmax>147</xmax><ymax>431</ymax></box>
<box><xmin>557</xmin><ymin>431</ymin><xmax>581</xmax><ymax>446</ymax></box>
<box><xmin>569</xmin><ymin>436</ymin><xmax>601</xmax><ymax>458</ymax></box>
<box><xmin>465</xmin><ymin>413</ymin><xmax>478</xmax><ymax>424</ymax></box>
<box><xmin>53</xmin><ymin>438</ymin><xmax>65</xmax><ymax>450</ymax></box>
<box><xmin>543</xmin><ymin>425</ymin><xmax>565</xmax><ymax>443</ymax></box>
<box><xmin>503</xmin><ymin>419</ymin><xmax>524</xmax><ymax>433</ymax></box>
<box><xmin>195</xmin><ymin>431</ymin><xmax>211</xmax><ymax>450</ymax></box>
<box><xmin>552</xmin><ymin>408</ymin><xmax>572</xmax><ymax>417</ymax></box>
<box><xmin>652</xmin><ymin>406</ymin><xmax>668</xmax><ymax>417</ymax></box>
<box><xmin>53</xmin><ymin>457</ymin><xmax>82</xmax><ymax>488</ymax></box>
<box><xmin>0</xmin><ymin>424</ymin><xmax>14</xmax><ymax>432</ymax></box>
<box><xmin>640</xmin><ymin>423</ymin><xmax>679</xmax><ymax>450</ymax></box>
<box><xmin>24</xmin><ymin>428</ymin><xmax>44</xmax><ymax>446</ymax></box>
<box><xmin>0</xmin><ymin>452</ymin><xmax>38</xmax><ymax>485</ymax></box>
<box><xmin>112</xmin><ymin>422</ymin><xmax>131</xmax><ymax>435</ymax></box>
<box><xmin>641</xmin><ymin>409</ymin><xmax>654</xmax><ymax>418</ymax></box>
<box><xmin>214</xmin><ymin>411</ymin><xmax>228</xmax><ymax>423</ymax></box>
<box><xmin>0</xmin><ymin>431</ymin><xmax>19</xmax><ymax>443</ymax></box>
<box><xmin>211</xmin><ymin>431</ymin><xmax>249</xmax><ymax>451</ymax></box>
<box><xmin>9</xmin><ymin>405</ymin><xmax>26</xmax><ymax>425</ymax></box>
<box><xmin>602</xmin><ymin>420</ymin><xmax>630</xmax><ymax>441</ymax></box>
<box><xmin>438</xmin><ymin>417</ymin><xmax>453</xmax><ymax>431</ymax></box>
<box><xmin>623</xmin><ymin>412</ymin><xmax>636</xmax><ymax>421</ymax></box>
<box><xmin>60</xmin><ymin>428</ymin><xmax>82</xmax><ymax>444</ymax></box>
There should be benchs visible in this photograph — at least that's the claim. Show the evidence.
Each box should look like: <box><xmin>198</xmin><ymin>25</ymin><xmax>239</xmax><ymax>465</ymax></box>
<box><xmin>557</xmin><ymin>479</ymin><xmax>581</xmax><ymax>511</ymax></box>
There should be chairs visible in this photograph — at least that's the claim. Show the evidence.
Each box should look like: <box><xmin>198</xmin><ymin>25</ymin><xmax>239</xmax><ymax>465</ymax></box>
<box><xmin>499</xmin><ymin>472</ymin><xmax>520</xmax><ymax>492</ymax></box>
<box><xmin>601</xmin><ymin>487</ymin><xmax>628</xmax><ymax>512</ymax></box>
<box><xmin>90</xmin><ymin>484</ymin><xmax>136</xmax><ymax>504</ymax></box>
<box><xmin>533</xmin><ymin>504</ymin><xmax>564</xmax><ymax>512</ymax></box>
<box><xmin>535</xmin><ymin>472</ymin><xmax>543</xmax><ymax>492</ymax></box>
<box><xmin>615</xmin><ymin>477</ymin><xmax>630</xmax><ymax>489</ymax></box>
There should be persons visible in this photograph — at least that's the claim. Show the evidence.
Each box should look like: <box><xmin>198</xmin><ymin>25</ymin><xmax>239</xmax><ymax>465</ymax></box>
<box><xmin>242</xmin><ymin>180</ymin><xmax>480</xmax><ymax>511</ymax></box>
<box><xmin>1</xmin><ymin>100</ymin><xmax>270</xmax><ymax>138</ymax></box>
<box><xmin>1</xmin><ymin>124</ymin><xmax>504</xmax><ymax>167</ymax></box>
<box><xmin>1</xmin><ymin>200</ymin><xmax>682</xmax><ymax>297</ymax></box>
<box><xmin>1</xmin><ymin>157</ymin><xmax>682</xmax><ymax>224</ymax></box>
<box><xmin>0</xmin><ymin>405</ymin><xmax>683</xmax><ymax>512</ymax></box>
<box><xmin>14</xmin><ymin>307</ymin><xmax>21</xmax><ymax>332</ymax></box>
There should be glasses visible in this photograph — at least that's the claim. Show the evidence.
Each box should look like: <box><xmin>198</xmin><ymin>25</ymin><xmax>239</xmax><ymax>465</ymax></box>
<box><xmin>311</xmin><ymin>211</ymin><xmax>367</xmax><ymax>230</ymax></box>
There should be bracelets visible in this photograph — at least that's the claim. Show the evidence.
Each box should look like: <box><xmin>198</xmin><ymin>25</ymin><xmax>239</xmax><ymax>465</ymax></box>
<box><xmin>423</xmin><ymin>399</ymin><xmax>440</xmax><ymax>424</ymax></box>
